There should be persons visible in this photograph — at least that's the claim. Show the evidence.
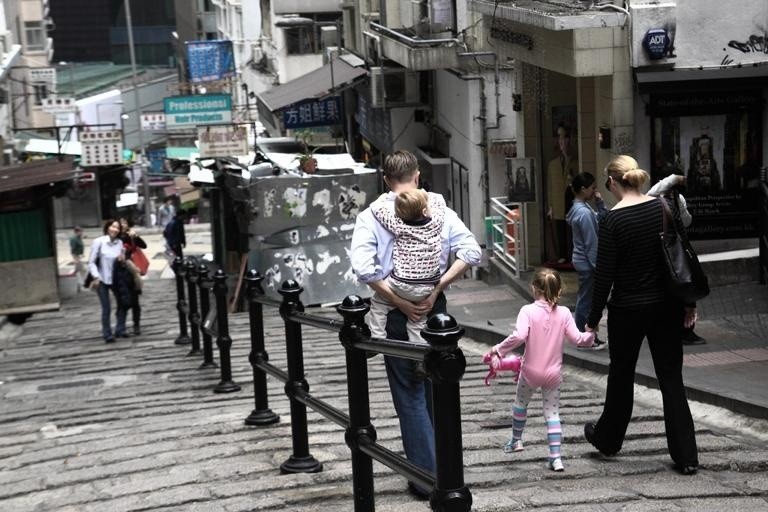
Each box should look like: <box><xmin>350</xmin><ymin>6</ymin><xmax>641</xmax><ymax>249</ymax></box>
<box><xmin>584</xmin><ymin>156</ymin><xmax>700</xmax><ymax>474</ymax></box>
<box><xmin>686</xmin><ymin>134</ymin><xmax>721</xmax><ymax>191</ymax></box>
<box><xmin>564</xmin><ymin>173</ymin><xmax>608</xmax><ymax>352</ymax></box>
<box><xmin>158</xmin><ymin>197</ymin><xmax>176</xmax><ymax>257</ymax></box>
<box><xmin>645</xmin><ymin>166</ymin><xmax>705</xmax><ymax>346</ymax></box>
<box><xmin>87</xmin><ymin>216</ymin><xmax>142</xmax><ymax>342</ymax></box>
<box><xmin>488</xmin><ymin>267</ymin><xmax>595</xmax><ymax>472</ymax></box>
<box><xmin>365</xmin><ymin>188</ymin><xmax>446</xmax><ymax>384</ymax></box>
<box><xmin>546</xmin><ymin>128</ymin><xmax>579</xmax><ymax>263</ymax></box>
<box><xmin>163</xmin><ymin>210</ymin><xmax>188</xmax><ymax>260</ymax></box>
<box><xmin>516</xmin><ymin>167</ymin><xmax>529</xmax><ymax>191</ymax></box>
<box><xmin>349</xmin><ymin>150</ymin><xmax>482</xmax><ymax>500</ymax></box>
<box><xmin>69</xmin><ymin>225</ymin><xmax>86</xmax><ymax>278</ymax></box>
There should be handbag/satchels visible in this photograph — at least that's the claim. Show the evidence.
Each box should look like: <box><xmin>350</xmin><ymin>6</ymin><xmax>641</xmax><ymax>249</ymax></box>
<box><xmin>661</xmin><ymin>230</ymin><xmax>712</xmax><ymax>304</ymax></box>
<box><xmin>83</xmin><ymin>255</ymin><xmax>101</xmax><ymax>289</ymax></box>
<box><xmin>129</xmin><ymin>247</ymin><xmax>150</xmax><ymax>276</ymax></box>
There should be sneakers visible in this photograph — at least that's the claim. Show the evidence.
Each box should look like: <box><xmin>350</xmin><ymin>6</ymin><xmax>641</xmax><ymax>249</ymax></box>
<box><xmin>407</xmin><ymin>481</ymin><xmax>431</xmax><ymax>502</ymax></box>
<box><xmin>684</xmin><ymin>330</ymin><xmax>710</xmax><ymax>347</ymax></box>
<box><xmin>503</xmin><ymin>439</ymin><xmax>525</xmax><ymax>455</ymax></box>
<box><xmin>584</xmin><ymin>422</ymin><xmax>617</xmax><ymax>459</ymax></box>
<box><xmin>576</xmin><ymin>335</ymin><xmax>608</xmax><ymax>351</ymax></box>
<box><xmin>412</xmin><ymin>360</ymin><xmax>432</xmax><ymax>381</ymax></box>
<box><xmin>367</xmin><ymin>348</ymin><xmax>384</xmax><ymax>360</ymax></box>
<box><xmin>546</xmin><ymin>457</ymin><xmax>566</xmax><ymax>473</ymax></box>
<box><xmin>104</xmin><ymin>330</ymin><xmax>141</xmax><ymax>344</ymax></box>
